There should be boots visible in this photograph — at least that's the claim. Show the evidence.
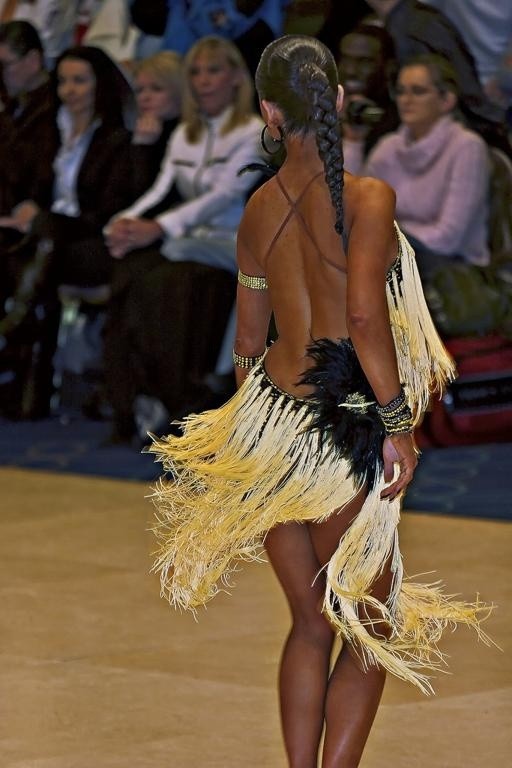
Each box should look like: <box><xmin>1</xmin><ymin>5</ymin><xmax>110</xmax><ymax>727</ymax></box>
<box><xmin>1</xmin><ymin>238</ymin><xmax>54</xmax><ymax>343</ymax></box>
<box><xmin>10</xmin><ymin>288</ymin><xmax>61</xmax><ymax>422</ymax></box>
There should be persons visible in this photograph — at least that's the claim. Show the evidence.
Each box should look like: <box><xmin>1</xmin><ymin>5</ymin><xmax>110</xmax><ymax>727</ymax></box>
<box><xmin>147</xmin><ymin>33</ymin><xmax>500</xmax><ymax>768</ymax></box>
<box><xmin>1</xmin><ymin>1</ymin><xmax>512</xmax><ymax>452</ymax></box>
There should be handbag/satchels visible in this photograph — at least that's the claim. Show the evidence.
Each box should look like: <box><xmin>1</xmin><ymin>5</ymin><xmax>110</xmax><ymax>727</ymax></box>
<box><xmin>415</xmin><ymin>333</ymin><xmax>511</xmax><ymax>449</ymax></box>
